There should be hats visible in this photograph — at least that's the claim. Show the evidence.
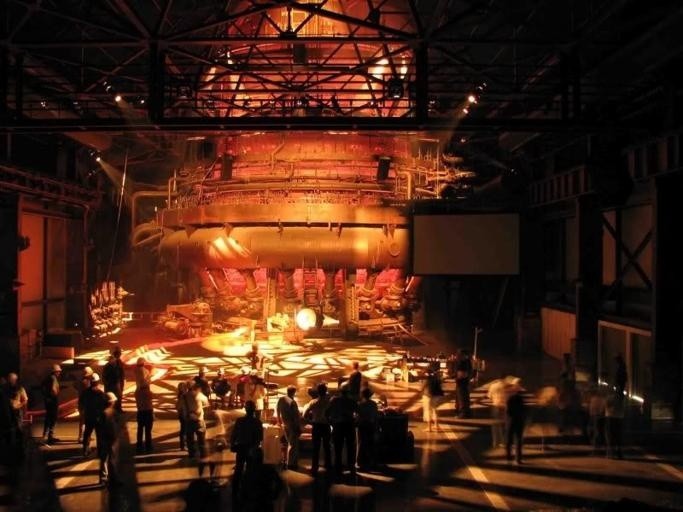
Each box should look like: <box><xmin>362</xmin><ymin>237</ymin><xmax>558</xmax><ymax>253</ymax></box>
<box><xmin>196</xmin><ymin>364</ymin><xmax>266</xmax><ymax>381</ymax></box>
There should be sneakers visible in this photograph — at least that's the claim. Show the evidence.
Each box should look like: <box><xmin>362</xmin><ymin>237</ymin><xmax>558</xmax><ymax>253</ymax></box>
<box><xmin>38</xmin><ymin>432</ymin><xmax>104</xmax><ymax>460</ymax></box>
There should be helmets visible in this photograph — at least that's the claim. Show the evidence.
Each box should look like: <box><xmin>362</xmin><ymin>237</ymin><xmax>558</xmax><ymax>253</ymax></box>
<box><xmin>89</xmin><ymin>373</ymin><xmax>101</xmax><ymax>382</ymax></box>
<box><xmin>49</xmin><ymin>364</ymin><xmax>62</xmax><ymax>373</ymax></box>
<box><xmin>81</xmin><ymin>367</ymin><xmax>94</xmax><ymax>377</ymax></box>
<box><xmin>102</xmin><ymin>391</ymin><xmax>119</xmax><ymax>403</ymax></box>
